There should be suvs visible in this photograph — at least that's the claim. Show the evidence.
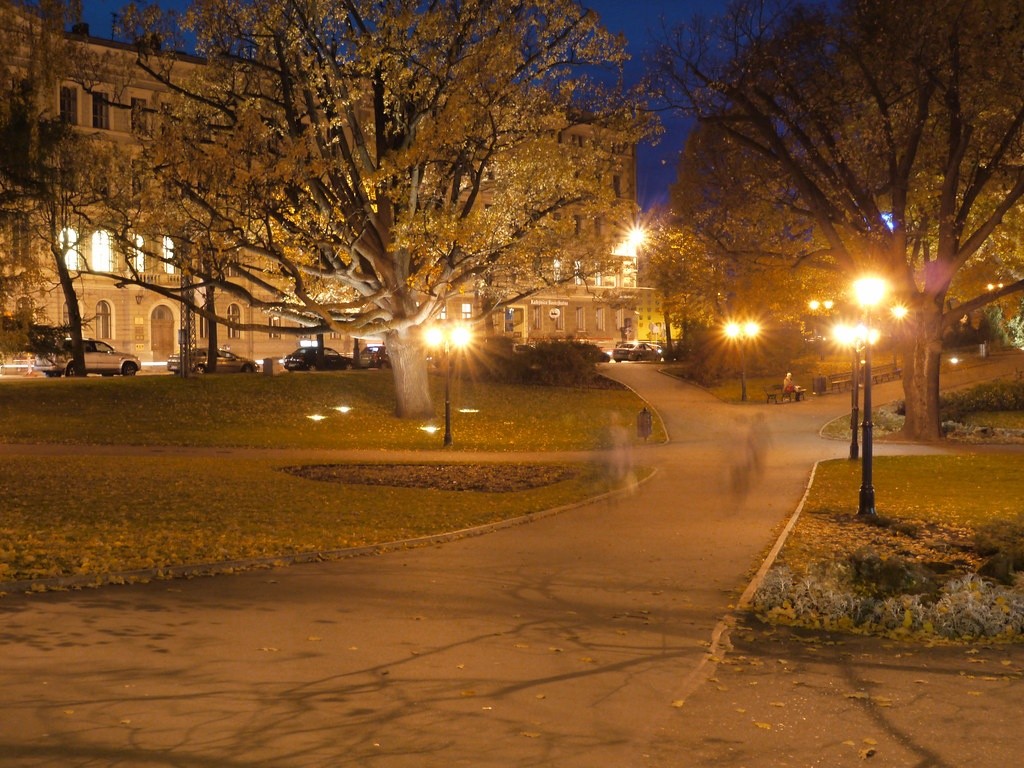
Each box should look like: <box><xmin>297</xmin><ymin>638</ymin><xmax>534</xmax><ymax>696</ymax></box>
<box><xmin>33</xmin><ymin>336</ymin><xmax>141</xmax><ymax>377</ymax></box>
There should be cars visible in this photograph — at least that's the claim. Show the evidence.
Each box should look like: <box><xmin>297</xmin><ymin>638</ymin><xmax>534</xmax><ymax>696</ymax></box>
<box><xmin>358</xmin><ymin>346</ymin><xmax>390</xmax><ymax>370</ymax></box>
<box><xmin>614</xmin><ymin>342</ymin><xmax>661</xmax><ymax>362</ymax></box>
<box><xmin>283</xmin><ymin>347</ymin><xmax>357</xmax><ymax>372</ymax></box>
<box><xmin>166</xmin><ymin>347</ymin><xmax>260</xmax><ymax>375</ymax></box>
<box><xmin>580</xmin><ymin>344</ymin><xmax>610</xmax><ymax>362</ymax></box>
<box><xmin>511</xmin><ymin>343</ymin><xmax>534</xmax><ymax>354</ymax></box>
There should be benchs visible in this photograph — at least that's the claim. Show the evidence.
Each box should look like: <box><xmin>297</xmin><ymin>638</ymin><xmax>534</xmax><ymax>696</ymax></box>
<box><xmin>870</xmin><ymin>363</ymin><xmax>903</xmax><ymax>384</ymax></box>
<box><xmin>764</xmin><ymin>384</ymin><xmax>807</xmax><ymax>404</ymax></box>
<box><xmin>829</xmin><ymin>372</ymin><xmax>853</xmax><ymax>393</ymax></box>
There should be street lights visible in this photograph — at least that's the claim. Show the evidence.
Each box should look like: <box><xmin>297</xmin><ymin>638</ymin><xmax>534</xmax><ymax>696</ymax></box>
<box><xmin>849</xmin><ymin>274</ymin><xmax>885</xmax><ymax>520</ymax></box>
<box><xmin>838</xmin><ymin>324</ymin><xmax>860</xmax><ymax>461</ymax></box>
<box><xmin>420</xmin><ymin>321</ymin><xmax>478</xmax><ymax>448</ymax></box>
<box><xmin>721</xmin><ymin>321</ymin><xmax>759</xmax><ymax>402</ymax></box>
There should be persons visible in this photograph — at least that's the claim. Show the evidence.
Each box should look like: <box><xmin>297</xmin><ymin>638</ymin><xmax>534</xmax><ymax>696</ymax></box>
<box><xmin>782</xmin><ymin>373</ymin><xmax>800</xmax><ymax>401</ymax></box>
